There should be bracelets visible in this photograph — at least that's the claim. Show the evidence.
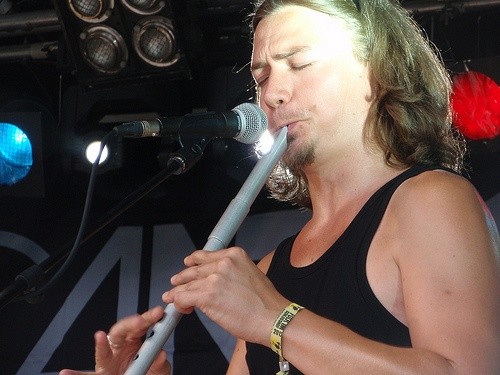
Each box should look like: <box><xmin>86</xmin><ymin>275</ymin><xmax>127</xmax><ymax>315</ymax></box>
<box><xmin>270</xmin><ymin>300</ymin><xmax>305</xmax><ymax>375</ymax></box>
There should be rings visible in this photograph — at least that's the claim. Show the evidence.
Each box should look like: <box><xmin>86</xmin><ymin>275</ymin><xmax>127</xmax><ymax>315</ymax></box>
<box><xmin>106</xmin><ymin>334</ymin><xmax>127</xmax><ymax>349</ymax></box>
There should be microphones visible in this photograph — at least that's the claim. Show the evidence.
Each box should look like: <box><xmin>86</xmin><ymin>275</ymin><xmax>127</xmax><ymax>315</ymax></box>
<box><xmin>113</xmin><ymin>103</ymin><xmax>268</xmax><ymax>144</ymax></box>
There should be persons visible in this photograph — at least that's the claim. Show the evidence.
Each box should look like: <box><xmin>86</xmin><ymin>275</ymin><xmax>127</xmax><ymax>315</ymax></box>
<box><xmin>57</xmin><ymin>0</ymin><xmax>499</xmax><ymax>375</ymax></box>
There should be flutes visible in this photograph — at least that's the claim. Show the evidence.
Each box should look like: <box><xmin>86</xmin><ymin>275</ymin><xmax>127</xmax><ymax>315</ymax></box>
<box><xmin>122</xmin><ymin>126</ymin><xmax>289</xmax><ymax>374</ymax></box>
